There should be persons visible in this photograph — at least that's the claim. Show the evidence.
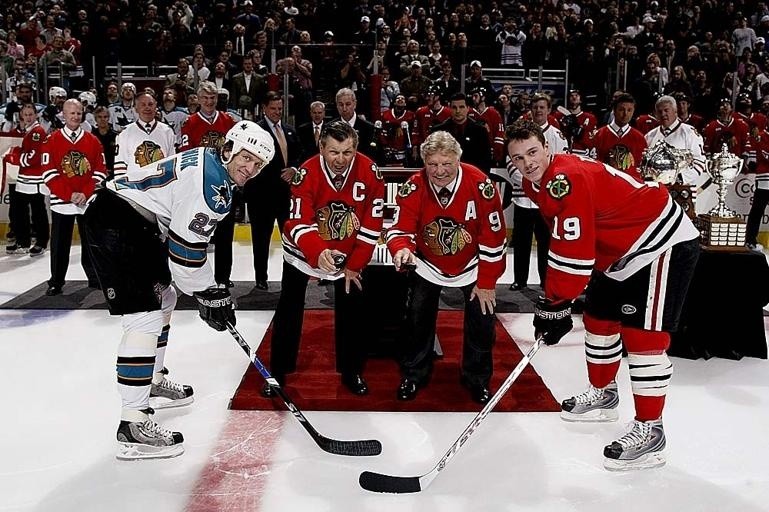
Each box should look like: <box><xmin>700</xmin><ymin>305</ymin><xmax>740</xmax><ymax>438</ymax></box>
<box><xmin>81</xmin><ymin>121</ymin><xmax>275</xmax><ymax>461</ymax></box>
<box><xmin>1</xmin><ymin>2</ymin><xmax>769</xmax><ymax>297</ymax></box>
<box><xmin>502</xmin><ymin>120</ymin><xmax>702</xmax><ymax>473</ymax></box>
<box><xmin>260</xmin><ymin>121</ymin><xmax>385</xmax><ymax>397</ymax></box>
<box><xmin>381</xmin><ymin>130</ymin><xmax>509</xmax><ymax>404</ymax></box>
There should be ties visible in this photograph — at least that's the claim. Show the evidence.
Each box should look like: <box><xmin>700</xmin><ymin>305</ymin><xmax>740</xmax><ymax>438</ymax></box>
<box><xmin>71</xmin><ymin>131</ymin><xmax>76</xmax><ymax>142</ymax></box>
<box><xmin>238</xmin><ymin>37</ymin><xmax>242</xmax><ymax>56</ymax></box>
<box><xmin>440</xmin><ymin>188</ymin><xmax>449</xmax><ymax>206</ymax></box>
<box><xmin>273</xmin><ymin>124</ymin><xmax>288</xmax><ymax>166</ymax></box>
<box><xmin>334</xmin><ymin>175</ymin><xmax>343</xmax><ymax>189</ymax></box>
<box><xmin>315</xmin><ymin>126</ymin><xmax>320</xmax><ymax>146</ymax></box>
<box><xmin>145</xmin><ymin>122</ymin><xmax>152</xmax><ymax>134</ymax></box>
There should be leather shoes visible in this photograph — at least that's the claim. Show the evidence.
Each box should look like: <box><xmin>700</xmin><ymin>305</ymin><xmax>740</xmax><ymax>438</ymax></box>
<box><xmin>257</xmin><ymin>280</ymin><xmax>269</xmax><ymax>290</ymax></box>
<box><xmin>470</xmin><ymin>383</ymin><xmax>492</xmax><ymax>404</ymax></box>
<box><xmin>45</xmin><ymin>287</ymin><xmax>64</xmax><ymax>296</ymax></box>
<box><xmin>397</xmin><ymin>376</ymin><xmax>420</xmax><ymax>400</ymax></box>
<box><xmin>263</xmin><ymin>373</ymin><xmax>285</xmax><ymax>398</ymax></box>
<box><xmin>510</xmin><ymin>282</ymin><xmax>527</xmax><ymax>290</ymax></box>
<box><xmin>342</xmin><ymin>374</ymin><xmax>369</xmax><ymax>396</ymax></box>
<box><xmin>88</xmin><ymin>279</ymin><xmax>98</xmax><ymax>288</ymax></box>
<box><xmin>215</xmin><ymin>279</ymin><xmax>234</xmax><ymax>288</ymax></box>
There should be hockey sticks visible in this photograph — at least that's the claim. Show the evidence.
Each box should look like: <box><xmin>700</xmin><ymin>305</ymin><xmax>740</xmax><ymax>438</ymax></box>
<box><xmin>359</xmin><ymin>332</ymin><xmax>549</xmax><ymax>493</ymax></box>
<box><xmin>225</xmin><ymin>319</ymin><xmax>382</xmax><ymax>456</ymax></box>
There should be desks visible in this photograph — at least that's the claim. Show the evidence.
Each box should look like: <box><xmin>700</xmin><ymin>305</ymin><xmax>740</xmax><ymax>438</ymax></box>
<box><xmin>672</xmin><ymin>243</ymin><xmax>769</xmax><ymax>358</ymax></box>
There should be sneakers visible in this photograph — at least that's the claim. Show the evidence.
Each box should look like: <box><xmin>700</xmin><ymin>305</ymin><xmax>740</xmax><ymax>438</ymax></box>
<box><xmin>116</xmin><ymin>420</ymin><xmax>184</xmax><ymax>446</ymax></box>
<box><xmin>562</xmin><ymin>380</ymin><xmax>619</xmax><ymax>413</ymax></box>
<box><xmin>29</xmin><ymin>245</ymin><xmax>43</xmax><ymax>254</ymax></box>
<box><xmin>604</xmin><ymin>418</ymin><xmax>666</xmax><ymax>460</ymax></box>
<box><xmin>149</xmin><ymin>378</ymin><xmax>194</xmax><ymax>401</ymax></box>
<box><xmin>6</xmin><ymin>241</ymin><xmax>31</xmax><ymax>251</ymax></box>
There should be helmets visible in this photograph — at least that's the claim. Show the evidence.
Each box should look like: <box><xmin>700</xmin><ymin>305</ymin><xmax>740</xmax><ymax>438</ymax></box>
<box><xmin>49</xmin><ymin>86</ymin><xmax>67</xmax><ymax>102</ymax></box>
<box><xmin>220</xmin><ymin>120</ymin><xmax>275</xmax><ymax>187</ymax></box>
<box><xmin>78</xmin><ymin>91</ymin><xmax>97</xmax><ymax>112</ymax></box>
<box><xmin>120</xmin><ymin>82</ymin><xmax>137</xmax><ymax>97</ymax></box>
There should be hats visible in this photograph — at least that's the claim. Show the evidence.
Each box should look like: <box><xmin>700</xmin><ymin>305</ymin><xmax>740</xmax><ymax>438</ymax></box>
<box><xmin>754</xmin><ymin>37</ymin><xmax>765</xmax><ymax>45</ymax></box>
<box><xmin>411</xmin><ymin>60</ymin><xmax>421</xmax><ymax>67</ymax></box>
<box><xmin>643</xmin><ymin>16</ymin><xmax>656</xmax><ymax>23</ymax></box>
<box><xmin>324</xmin><ymin>31</ymin><xmax>334</xmax><ymax>36</ymax></box>
<box><xmin>284</xmin><ymin>6</ymin><xmax>299</xmax><ymax>15</ymax></box>
<box><xmin>470</xmin><ymin>60</ymin><xmax>481</xmax><ymax>68</ymax></box>
<box><xmin>584</xmin><ymin>18</ymin><xmax>594</xmax><ymax>25</ymax></box>
<box><xmin>361</xmin><ymin>16</ymin><xmax>370</xmax><ymax>24</ymax></box>
<box><xmin>218</xmin><ymin>87</ymin><xmax>229</xmax><ymax>100</ymax></box>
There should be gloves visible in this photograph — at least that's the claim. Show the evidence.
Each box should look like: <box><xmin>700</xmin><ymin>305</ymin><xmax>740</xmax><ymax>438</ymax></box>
<box><xmin>533</xmin><ymin>296</ymin><xmax>574</xmax><ymax>346</ymax></box>
<box><xmin>193</xmin><ymin>289</ymin><xmax>236</xmax><ymax>331</ymax></box>
